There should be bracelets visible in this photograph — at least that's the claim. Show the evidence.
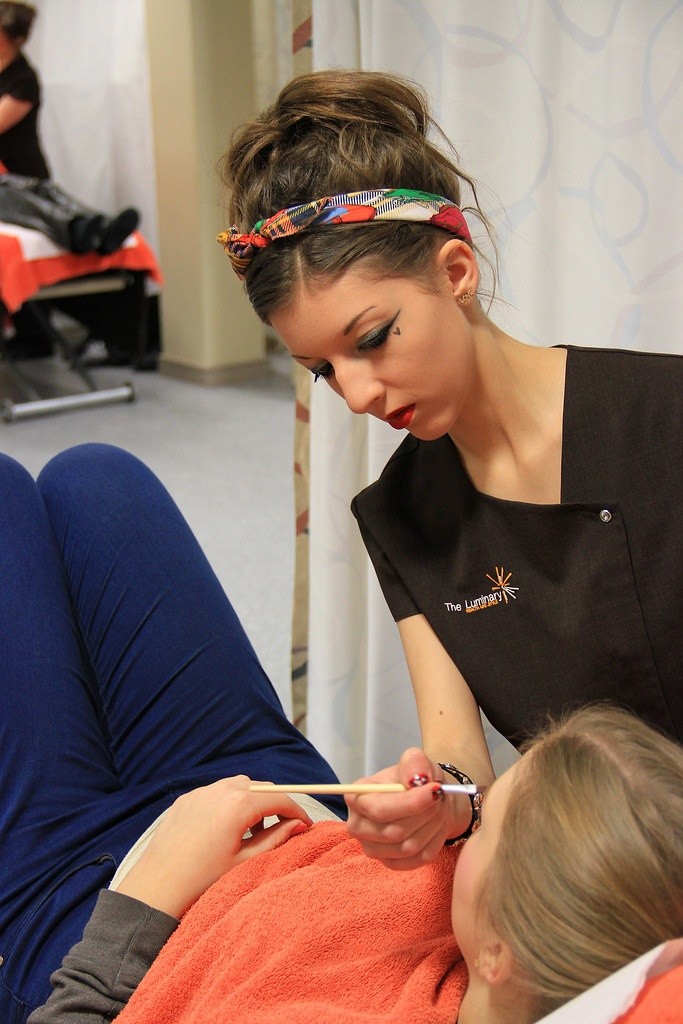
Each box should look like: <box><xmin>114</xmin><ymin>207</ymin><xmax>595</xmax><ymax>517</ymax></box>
<box><xmin>436</xmin><ymin>761</ymin><xmax>483</xmax><ymax>849</ymax></box>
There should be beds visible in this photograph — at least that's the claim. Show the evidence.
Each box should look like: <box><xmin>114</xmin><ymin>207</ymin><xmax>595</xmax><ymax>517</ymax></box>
<box><xmin>0</xmin><ymin>160</ymin><xmax>165</xmax><ymax>422</ymax></box>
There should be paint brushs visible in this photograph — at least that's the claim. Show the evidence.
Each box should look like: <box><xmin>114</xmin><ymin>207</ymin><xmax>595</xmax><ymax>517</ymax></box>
<box><xmin>248</xmin><ymin>784</ymin><xmax>490</xmax><ymax>795</ymax></box>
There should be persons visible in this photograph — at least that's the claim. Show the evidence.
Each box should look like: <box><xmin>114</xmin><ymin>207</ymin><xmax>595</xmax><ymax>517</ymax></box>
<box><xmin>0</xmin><ymin>440</ymin><xmax>682</xmax><ymax>1023</ymax></box>
<box><xmin>215</xmin><ymin>71</ymin><xmax>683</xmax><ymax>869</ymax></box>
<box><xmin>0</xmin><ymin>160</ymin><xmax>141</xmax><ymax>256</ymax></box>
<box><xmin>0</xmin><ymin>0</ymin><xmax>51</xmax><ymax>181</ymax></box>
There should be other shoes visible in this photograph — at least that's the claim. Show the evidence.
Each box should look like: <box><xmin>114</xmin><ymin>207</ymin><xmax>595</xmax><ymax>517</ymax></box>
<box><xmin>99</xmin><ymin>207</ymin><xmax>139</xmax><ymax>254</ymax></box>
<box><xmin>68</xmin><ymin>212</ymin><xmax>105</xmax><ymax>254</ymax></box>
